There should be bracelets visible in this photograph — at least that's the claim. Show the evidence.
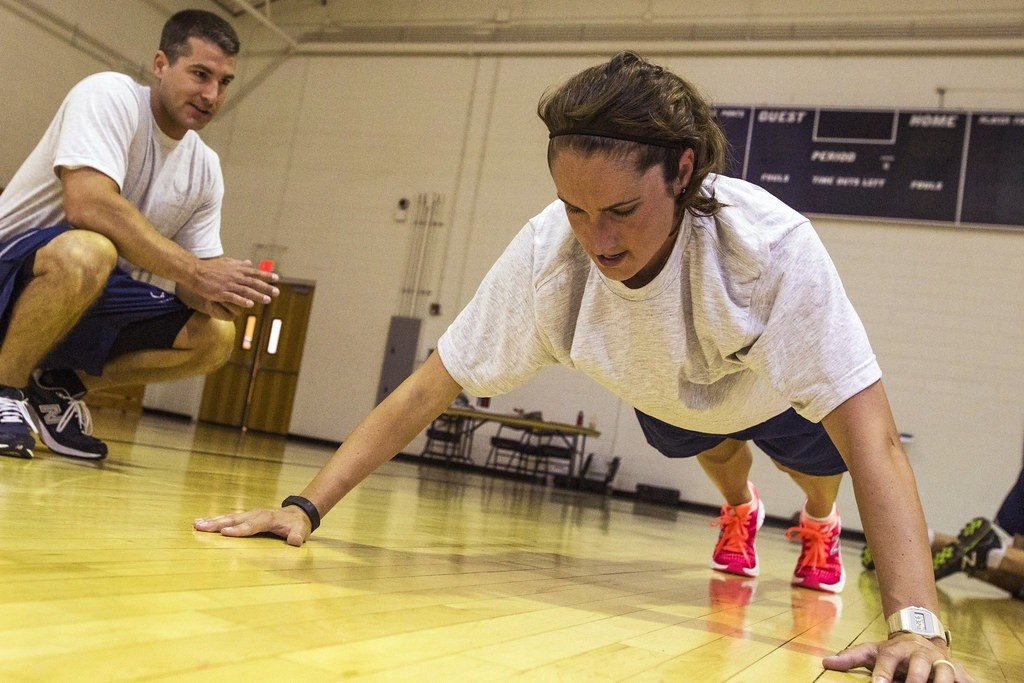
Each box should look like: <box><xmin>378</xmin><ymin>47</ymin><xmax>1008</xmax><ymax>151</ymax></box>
<box><xmin>282</xmin><ymin>495</ymin><xmax>320</xmax><ymax>533</ymax></box>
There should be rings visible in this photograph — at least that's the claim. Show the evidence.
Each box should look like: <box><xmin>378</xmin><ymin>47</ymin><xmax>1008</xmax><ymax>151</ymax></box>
<box><xmin>932</xmin><ymin>660</ymin><xmax>956</xmax><ymax>675</ymax></box>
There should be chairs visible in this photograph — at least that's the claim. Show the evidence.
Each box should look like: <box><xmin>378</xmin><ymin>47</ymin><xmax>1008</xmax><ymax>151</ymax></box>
<box><xmin>420</xmin><ymin>390</ymin><xmax>580</xmax><ymax>493</ymax></box>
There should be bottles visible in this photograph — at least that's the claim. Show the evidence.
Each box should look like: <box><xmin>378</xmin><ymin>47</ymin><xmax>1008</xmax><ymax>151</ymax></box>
<box><xmin>577</xmin><ymin>411</ymin><xmax>583</xmax><ymax>426</ymax></box>
<box><xmin>588</xmin><ymin>412</ymin><xmax>597</xmax><ymax>430</ymax></box>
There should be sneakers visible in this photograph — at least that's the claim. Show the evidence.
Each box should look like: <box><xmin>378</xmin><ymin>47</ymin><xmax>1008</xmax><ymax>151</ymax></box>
<box><xmin>786</xmin><ymin>495</ymin><xmax>846</xmax><ymax>592</ymax></box>
<box><xmin>709</xmin><ymin>480</ymin><xmax>765</xmax><ymax>576</ymax></box>
<box><xmin>861</xmin><ymin>544</ymin><xmax>876</xmax><ymax>572</ymax></box>
<box><xmin>0</xmin><ymin>385</ymin><xmax>38</xmax><ymax>459</ymax></box>
<box><xmin>932</xmin><ymin>516</ymin><xmax>1002</xmax><ymax>581</ymax></box>
<box><xmin>23</xmin><ymin>371</ymin><xmax>108</xmax><ymax>459</ymax></box>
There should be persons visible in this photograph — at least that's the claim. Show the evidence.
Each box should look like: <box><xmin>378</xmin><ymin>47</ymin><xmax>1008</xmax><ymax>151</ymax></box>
<box><xmin>0</xmin><ymin>9</ymin><xmax>279</xmax><ymax>459</ymax></box>
<box><xmin>860</xmin><ymin>462</ymin><xmax>1024</xmax><ymax>599</ymax></box>
<box><xmin>193</xmin><ymin>49</ymin><xmax>975</xmax><ymax>683</ymax></box>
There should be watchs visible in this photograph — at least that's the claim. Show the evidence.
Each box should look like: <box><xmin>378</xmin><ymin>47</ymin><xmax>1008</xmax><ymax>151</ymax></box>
<box><xmin>886</xmin><ymin>605</ymin><xmax>952</xmax><ymax>652</ymax></box>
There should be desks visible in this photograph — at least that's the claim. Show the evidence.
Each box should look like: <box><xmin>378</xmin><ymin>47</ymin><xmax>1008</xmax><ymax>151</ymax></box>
<box><xmin>442</xmin><ymin>408</ymin><xmax>601</xmax><ymax>493</ymax></box>
